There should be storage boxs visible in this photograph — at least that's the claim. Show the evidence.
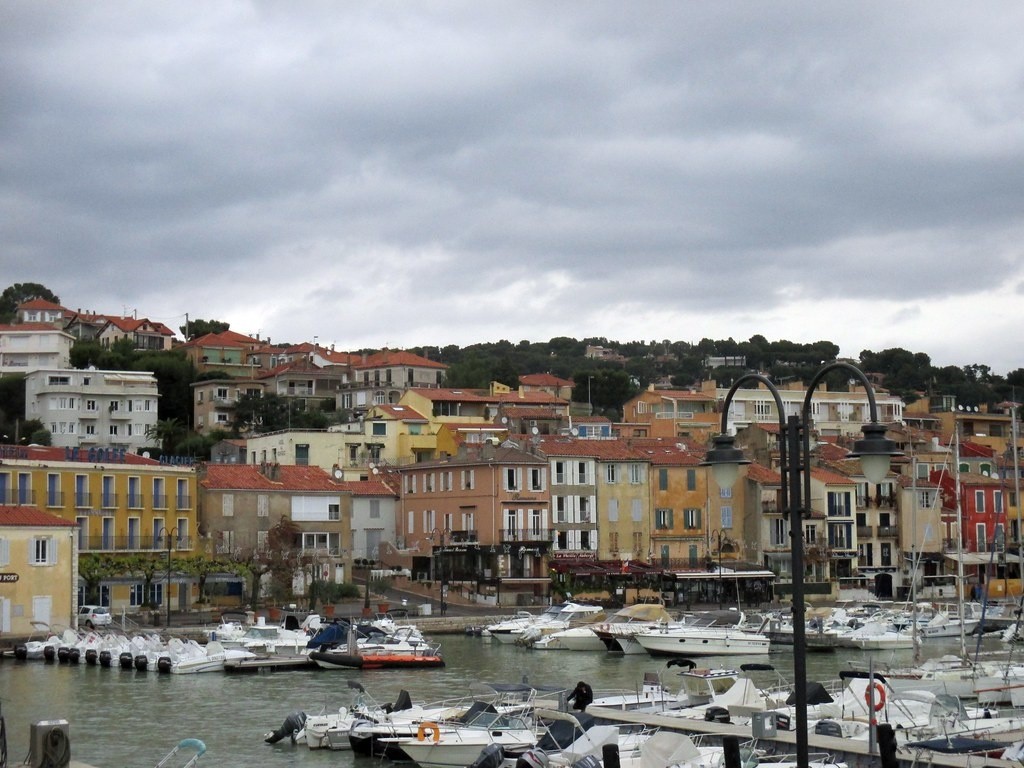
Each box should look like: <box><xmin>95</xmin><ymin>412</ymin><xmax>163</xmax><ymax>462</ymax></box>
<box><xmin>752</xmin><ymin>711</ymin><xmax>776</xmax><ymax>737</ymax></box>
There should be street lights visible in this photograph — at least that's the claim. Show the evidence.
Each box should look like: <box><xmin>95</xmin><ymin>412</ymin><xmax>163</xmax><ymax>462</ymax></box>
<box><xmin>711</xmin><ymin>526</ymin><xmax>727</xmax><ymax>611</ymax></box>
<box><xmin>429</xmin><ymin>527</ymin><xmax>454</xmax><ymax>616</ymax></box>
<box><xmin>154</xmin><ymin>523</ymin><xmax>182</xmax><ymax>625</ymax></box>
<box><xmin>712</xmin><ymin>358</ymin><xmax>906</xmax><ymax>767</ymax></box>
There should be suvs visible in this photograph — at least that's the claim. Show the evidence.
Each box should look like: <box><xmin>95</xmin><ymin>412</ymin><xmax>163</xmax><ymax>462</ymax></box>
<box><xmin>78</xmin><ymin>606</ymin><xmax>113</xmax><ymax>629</ymax></box>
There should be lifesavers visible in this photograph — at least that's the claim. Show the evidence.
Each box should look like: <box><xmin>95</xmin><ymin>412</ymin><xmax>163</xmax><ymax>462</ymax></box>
<box><xmin>418</xmin><ymin>723</ymin><xmax>439</xmax><ymax>741</ymax></box>
<box><xmin>865</xmin><ymin>682</ymin><xmax>886</xmax><ymax>711</ymax></box>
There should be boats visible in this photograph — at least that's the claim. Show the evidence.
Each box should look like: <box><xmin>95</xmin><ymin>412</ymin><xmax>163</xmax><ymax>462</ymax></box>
<box><xmin>4</xmin><ymin>598</ymin><xmax>258</xmax><ymax>676</ymax></box>
<box><xmin>204</xmin><ymin>606</ymin><xmax>249</xmax><ymax>644</ymax></box>
<box><xmin>481</xmin><ymin>568</ymin><xmax>1023</xmax><ymax>653</ymax></box>
<box><xmin>320</xmin><ymin>628</ymin><xmax>442</xmax><ymax>671</ymax></box>
<box><xmin>221</xmin><ymin>614</ymin><xmax>309</xmax><ymax>658</ymax></box>
<box><xmin>297</xmin><ymin>654</ymin><xmax>1024</xmax><ymax>768</ymax></box>
<box><xmin>286</xmin><ymin>608</ymin><xmax>424</xmax><ymax>651</ymax></box>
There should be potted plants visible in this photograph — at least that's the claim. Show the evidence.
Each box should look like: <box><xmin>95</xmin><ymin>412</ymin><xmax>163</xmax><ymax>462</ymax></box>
<box><xmin>314</xmin><ymin>579</ymin><xmax>339</xmax><ymax>616</ymax></box>
<box><xmin>263</xmin><ymin>579</ymin><xmax>289</xmax><ymax>622</ymax></box>
<box><xmin>372</xmin><ymin>578</ymin><xmax>390</xmax><ymax>612</ymax></box>
<box><xmin>207</xmin><ymin>580</ymin><xmax>229</xmax><ymax>624</ymax></box>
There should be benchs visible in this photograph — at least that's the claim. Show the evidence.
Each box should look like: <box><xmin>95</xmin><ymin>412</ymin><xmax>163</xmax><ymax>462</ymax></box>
<box><xmin>509</xmin><ymin>717</ymin><xmax>533</xmax><ymax>730</ymax></box>
